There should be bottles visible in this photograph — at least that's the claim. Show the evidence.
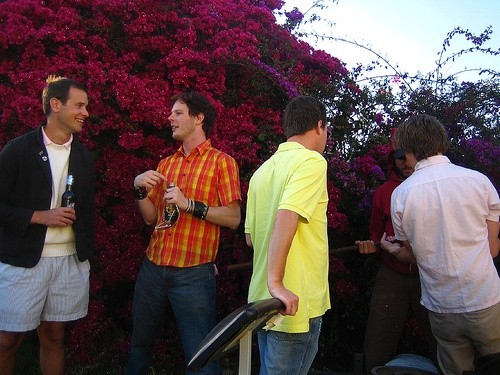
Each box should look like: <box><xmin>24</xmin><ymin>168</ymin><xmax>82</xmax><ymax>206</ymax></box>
<box><xmin>61</xmin><ymin>172</ymin><xmax>76</xmax><ymax>217</ymax></box>
<box><xmin>163</xmin><ymin>182</ymin><xmax>181</xmax><ymax>225</ymax></box>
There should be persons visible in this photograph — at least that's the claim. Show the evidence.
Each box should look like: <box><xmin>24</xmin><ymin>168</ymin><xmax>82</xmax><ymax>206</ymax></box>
<box><xmin>0</xmin><ymin>75</ymin><xmax>97</xmax><ymax>375</ymax></box>
<box><xmin>126</xmin><ymin>92</ymin><xmax>241</xmax><ymax>375</ymax></box>
<box><xmin>245</xmin><ymin>95</ymin><xmax>331</xmax><ymax>375</ymax></box>
<box><xmin>381</xmin><ymin>114</ymin><xmax>500</xmax><ymax>375</ymax></box>
<box><xmin>356</xmin><ymin>129</ymin><xmax>438</xmax><ymax>375</ymax></box>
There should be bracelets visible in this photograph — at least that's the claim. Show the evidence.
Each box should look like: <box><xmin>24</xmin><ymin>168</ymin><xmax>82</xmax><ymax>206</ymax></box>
<box><xmin>185</xmin><ymin>199</ymin><xmax>192</xmax><ymax>213</ymax></box>
<box><xmin>189</xmin><ymin>199</ymin><xmax>195</xmax><ymax>214</ymax></box>
<box><xmin>192</xmin><ymin>201</ymin><xmax>209</xmax><ymax>221</ymax></box>
<box><xmin>132</xmin><ymin>187</ymin><xmax>148</xmax><ymax>200</ymax></box>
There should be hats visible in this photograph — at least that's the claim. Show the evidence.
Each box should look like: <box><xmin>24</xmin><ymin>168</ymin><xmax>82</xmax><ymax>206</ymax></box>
<box><xmin>393</xmin><ymin>149</ymin><xmax>405</xmax><ymax>158</ymax></box>
